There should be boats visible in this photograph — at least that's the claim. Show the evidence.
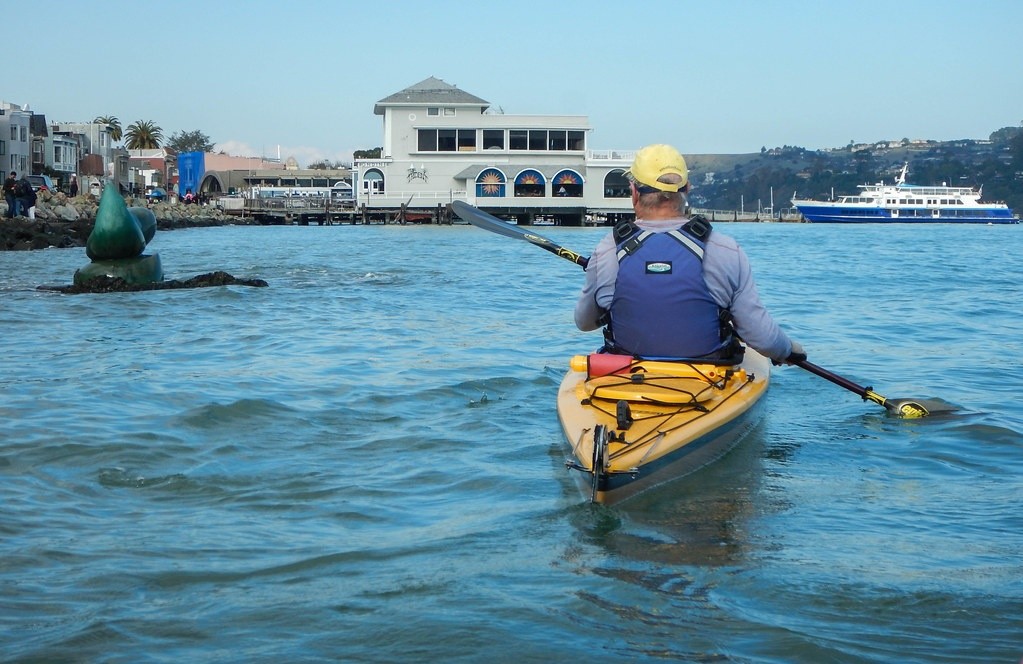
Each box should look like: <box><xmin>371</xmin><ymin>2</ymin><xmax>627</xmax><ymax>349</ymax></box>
<box><xmin>557</xmin><ymin>340</ymin><xmax>772</xmax><ymax>510</ymax></box>
<box><xmin>790</xmin><ymin>162</ymin><xmax>1020</xmax><ymax>224</ymax></box>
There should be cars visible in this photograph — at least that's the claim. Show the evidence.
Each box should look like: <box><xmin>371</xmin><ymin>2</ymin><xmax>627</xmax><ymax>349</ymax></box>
<box><xmin>26</xmin><ymin>175</ymin><xmax>54</xmax><ymax>193</ymax></box>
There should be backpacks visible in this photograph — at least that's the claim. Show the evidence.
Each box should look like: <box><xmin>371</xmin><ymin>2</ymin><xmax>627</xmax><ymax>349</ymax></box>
<box><xmin>14</xmin><ymin>181</ymin><xmax>25</xmax><ymax>196</ymax></box>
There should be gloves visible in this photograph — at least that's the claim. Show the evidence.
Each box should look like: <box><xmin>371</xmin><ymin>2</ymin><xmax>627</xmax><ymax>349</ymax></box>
<box><xmin>771</xmin><ymin>341</ymin><xmax>807</xmax><ymax>366</ymax></box>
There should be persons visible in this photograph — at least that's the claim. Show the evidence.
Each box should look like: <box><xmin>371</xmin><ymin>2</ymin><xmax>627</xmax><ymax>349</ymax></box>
<box><xmin>185</xmin><ymin>191</ymin><xmax>203</xmax><ymax>204</ymax></box>
<box><xmin>574</xmin><ymin>144</ymin><xmax>807</xmax><ymax>367</ymax></box>
<box><xmin>3</xmin><ymin>171</ymin><xmax>36</xmax><ymax>220</ymax></box>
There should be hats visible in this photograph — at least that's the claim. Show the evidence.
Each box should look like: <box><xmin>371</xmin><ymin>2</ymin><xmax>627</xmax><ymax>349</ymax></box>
<box><xmin>622</xmin><ymin>143</ymin><xmax>689</xmax><ymax>193</ymax></box>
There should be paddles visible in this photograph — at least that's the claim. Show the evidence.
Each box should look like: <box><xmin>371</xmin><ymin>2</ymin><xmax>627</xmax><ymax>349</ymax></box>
<box><xmin>451</xmin><ymin>200</ymin><xmax>960</xmax><ymax>419</ymax></box>
